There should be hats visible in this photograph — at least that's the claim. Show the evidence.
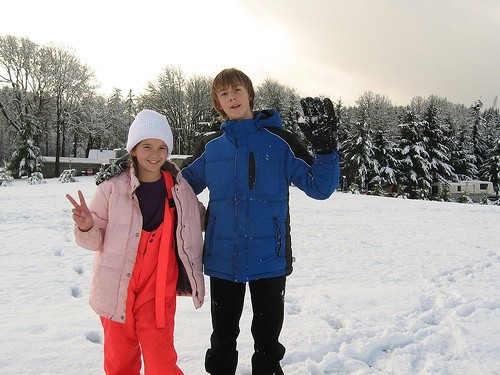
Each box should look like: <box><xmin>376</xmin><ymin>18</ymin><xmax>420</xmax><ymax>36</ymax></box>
<box><xmin>126</xmin><ymin>109</ymin><xmax>173</xmax><ymax>158</ymax></box>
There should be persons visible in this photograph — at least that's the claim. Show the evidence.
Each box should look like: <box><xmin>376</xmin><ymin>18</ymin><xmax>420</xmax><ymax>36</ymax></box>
<box><xmin>66</xmin><ymin>109</ymin><xmax>206</xmax><ymax>375</ymax></box>
<box><xmin>183</xmin><ymin>69</ymin><xmax>339</xmax><ymax>375</ymax></box>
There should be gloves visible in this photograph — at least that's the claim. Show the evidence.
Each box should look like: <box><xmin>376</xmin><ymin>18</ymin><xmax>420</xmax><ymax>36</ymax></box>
<box><xmin>96</xmin><ymin>154</ymin><xmax>132</xmax><ymax>185</ymax></box>
<box><xmin>296</xmin><ymin>97</ymin><xmax>337</xmax><ymax>154</ymax></box>
